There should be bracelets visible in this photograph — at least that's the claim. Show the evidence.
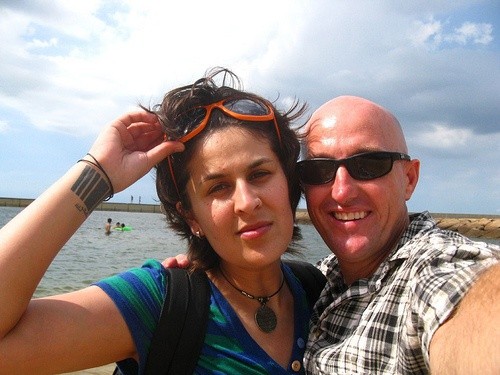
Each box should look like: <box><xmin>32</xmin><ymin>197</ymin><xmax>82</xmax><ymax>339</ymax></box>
<box><xmin>75</xmin><ymin>154</ymin><xmax>115</xmax><ymax>202</ymax></box>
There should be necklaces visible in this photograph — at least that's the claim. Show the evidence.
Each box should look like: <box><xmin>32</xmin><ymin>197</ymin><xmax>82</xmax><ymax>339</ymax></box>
<box><xmin>216</xmin><ymin>264</ymin><xmax>286</xmax><ymax>333</ymax></box>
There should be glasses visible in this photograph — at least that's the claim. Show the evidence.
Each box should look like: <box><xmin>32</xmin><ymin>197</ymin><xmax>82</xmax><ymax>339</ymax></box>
<box><xmin>291</xmin><ymin>150</ymin><xmax>410</xmax><ymax>186</ymax></box>
<box><xmin>162</xmin><ymin>93</ymin><xmax>285</xmax><ymax>203</ymax></box>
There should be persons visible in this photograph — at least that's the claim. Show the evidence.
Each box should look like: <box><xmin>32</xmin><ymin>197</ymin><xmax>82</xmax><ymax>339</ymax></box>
<box><xmin>105</xmin><ymin>218</ymin><xmax>125</xmax><ymax>232</ymax></box>
<box><xmin>0</xmin><ymin>67</ymin><xmax>328</xmax><ymax>375</ymax></box>
<box><xmin>302</xmin><ymin>96</ymin><xmax>500</xmax><ymax>375</ymax></box>
